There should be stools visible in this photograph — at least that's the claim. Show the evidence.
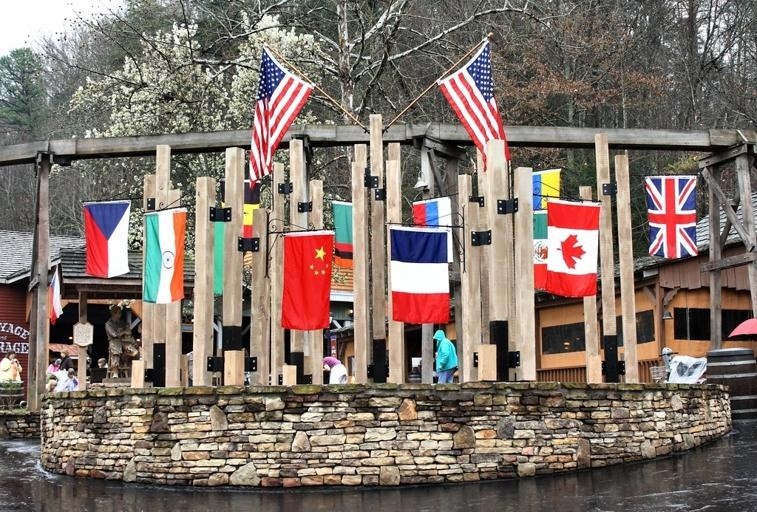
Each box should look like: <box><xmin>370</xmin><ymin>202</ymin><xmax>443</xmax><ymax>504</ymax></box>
<box><xmin>105</xmin><ymin>345</ymin><xmax>130</xmax><ymax>378</ymax></box>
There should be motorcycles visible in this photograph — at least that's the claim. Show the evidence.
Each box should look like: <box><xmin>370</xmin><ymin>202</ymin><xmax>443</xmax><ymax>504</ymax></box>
<box><xmin>648</xmin><ymin>346</ymin><xmax>709</xmax><ymax>386</ymax></box>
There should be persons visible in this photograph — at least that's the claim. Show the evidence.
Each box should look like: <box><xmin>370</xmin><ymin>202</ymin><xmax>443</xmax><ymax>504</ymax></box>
<box><xmin>431</xmin><ymin>329</ymin><xmax>458</xmax><ymax>384</ymax></box>
<box><xmin>45</xmin><ymin>374</ymin><xmax>58</xmax><ymax>392</ymax></box>
<box><xmin>0</xmin><ymin>350</ymin><xmax>23</xmax><ymax>382</ymax></box>
<box><xmin>47</xmin><ymin>358</ymin><xmax>61</xmax><ymax>372</ymax></box>
<box><xmin>62</xmin><ymin>368</ymin><xmax>77</xmax><ymax>391</ymax></box>
<box><xmin>105</xmin><ymin>305</ymin><xmax>131</xmax><ymax>378</ymax></box>
<box><xmin>90</xmin><ymin>357</ymin><xmax>108</xmax><ymax>384</ymax></box>
<box><xmin>59</xmin><ymin>349</ymin><xmax>73</xmax><ymax>370</ymax></box>
<box><xmin>322</xmin><ymin>356</ymin><xmax>348</xmax><ymax>385</ymax></box>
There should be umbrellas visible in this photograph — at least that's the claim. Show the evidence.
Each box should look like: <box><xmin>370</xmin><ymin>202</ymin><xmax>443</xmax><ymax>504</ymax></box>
<box><xmin>727</xmin><ymin>318</ymin><xmax>756</xmax><ymax>338</ymax></box>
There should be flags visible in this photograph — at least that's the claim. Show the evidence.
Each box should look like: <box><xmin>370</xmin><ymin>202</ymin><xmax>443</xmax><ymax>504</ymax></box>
<box><xmin>531</xmin><ymin>168</ymin><xmax>560</xmax><ymax>211</ymax></box>
<box><xmin>330</xmin><ymin>200</ymin><xmax>354</xmax><ymax>269</ymax></box>
<box><xmin>211</xmin><ymin>221</ymin><xmax>224</xmax><ymax>297</ymax></box>
<box><xmin>544</xmin><ymin>198</ymin><xmax>598</xmax><ymax>300</ymax></box>
<box><xmin>436</xmin><ymin>38</ymin><xmax>512</xmax><ymax>169</ymax></box>
<box><xmin>280</xmin><ymin>228</ymin><xmax>334</xmax><ymax>333</ymax></box>
<box><xmin>410</xmin><ymin>197</ymin><xmax>453</xmax><ymax>263</ymax></box>
<box><xmin>219</xmin><ymin>178</ymin><xmax>261</xmax><ymax>252</ymax></box>
<box><xmin>141</xmin><ymin>207</ymin><xmax>185</xmax><ymax>305</ymax></box>
<box><xmin>384</xmin><ymin>225</ymin><xmax>450</xmax><ymax>328</ymax></box>
<box><xmin>531</xmin><ymin>213</ymin><xmax>547</xmax><ymax>292</ymax></box>
<box><xmin>83</xmin><ymin>200</ymin><xmax>130</xmax><ymax>279</ymax></box>
<box><xmin>246</xmin><ymin>44</ymin><xmax>314</xmax><ymax>187</ymax></box>
<box><xmin>643</xmin><ymin>174</ymin><xmax>698</xmax><ymax>262</ymax></box>
